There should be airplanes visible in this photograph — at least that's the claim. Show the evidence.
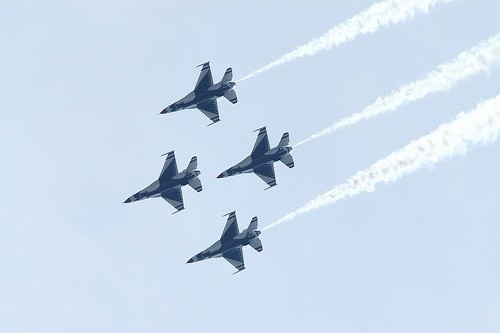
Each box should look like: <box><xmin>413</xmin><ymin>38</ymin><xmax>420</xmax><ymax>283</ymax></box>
<box><xmin>187</xmin><ymin>211</ymin><xmax>265</xmax><ymax>273</ymax></box>
<box><xmin>215</xmin><ymin>125</ymin><xmax>295</xmax><ymax>187</ymax></box>
<box><xmin>124</xmin><ymin>151</ymin><xmax>203</xmax><ymax>211</ymax></box>
<box><xmin>160</xmin><ymin>61</ymin><xmax>239</xmax><ymax>125</ymax></box>
